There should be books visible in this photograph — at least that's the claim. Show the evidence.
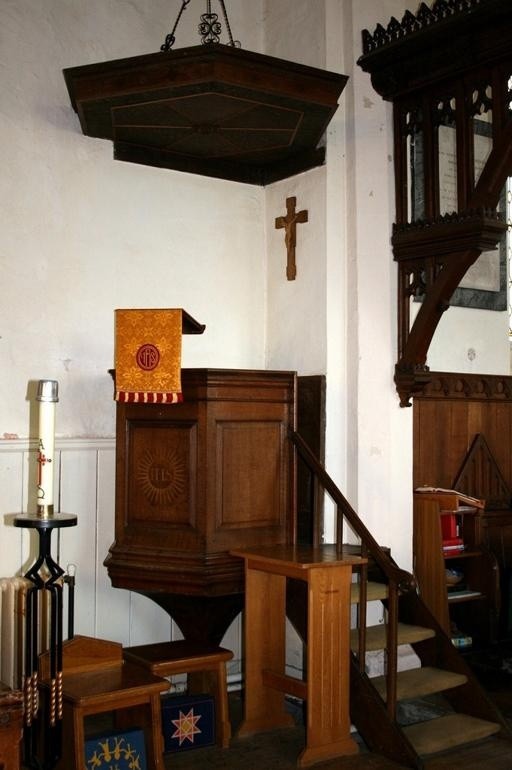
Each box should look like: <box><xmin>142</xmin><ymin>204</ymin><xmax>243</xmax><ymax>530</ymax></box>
<box><xmin>442</xmin><ymin>538</ymin><xmax>463</xmax><ymax>547</ymax></box>
<box><xmin>416</xmin><ymin>484</ymin><xmax>480</xmax><ymax>504</ymax></box>
<box><xmin>443</xmin><ymin>544</ymin><xmax>466</xmax><ymax>551</ymax></box>
<box><xmin>441</xmin><ymin>514</ymin><xmax>457</xmax><ymax>540</ymax></box>
<box><xmin>446</xmin><ymin>589</ymin><xmax>483</xmax><ymax>600</ymax></box>
<box><xmin>441</xmin><ymin>505</ymin><xmax>477</xmax><ymax>513</ymax></box>
<box><xmin>450</xmin><ymin>629</ymin><xmax>473</xmax><ymax>648</ymax></box>
<box><xmin>442</xmin><ymin>549</ymin><xmax>464</xmax><ymax>558</ymax></box>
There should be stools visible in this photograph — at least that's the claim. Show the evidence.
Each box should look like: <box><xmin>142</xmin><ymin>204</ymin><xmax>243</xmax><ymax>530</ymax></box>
<box><xmin>38</xmin><ymin>641</ymin><xmax>232</xmax><ymax>770</ymax></box>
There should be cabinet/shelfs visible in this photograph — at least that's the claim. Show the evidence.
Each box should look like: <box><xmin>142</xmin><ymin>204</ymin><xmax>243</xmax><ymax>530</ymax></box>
<box><xmin>416</xmin><ymin>495</ymin><xmax>512</xmax><ymax>684</ymax></box>
